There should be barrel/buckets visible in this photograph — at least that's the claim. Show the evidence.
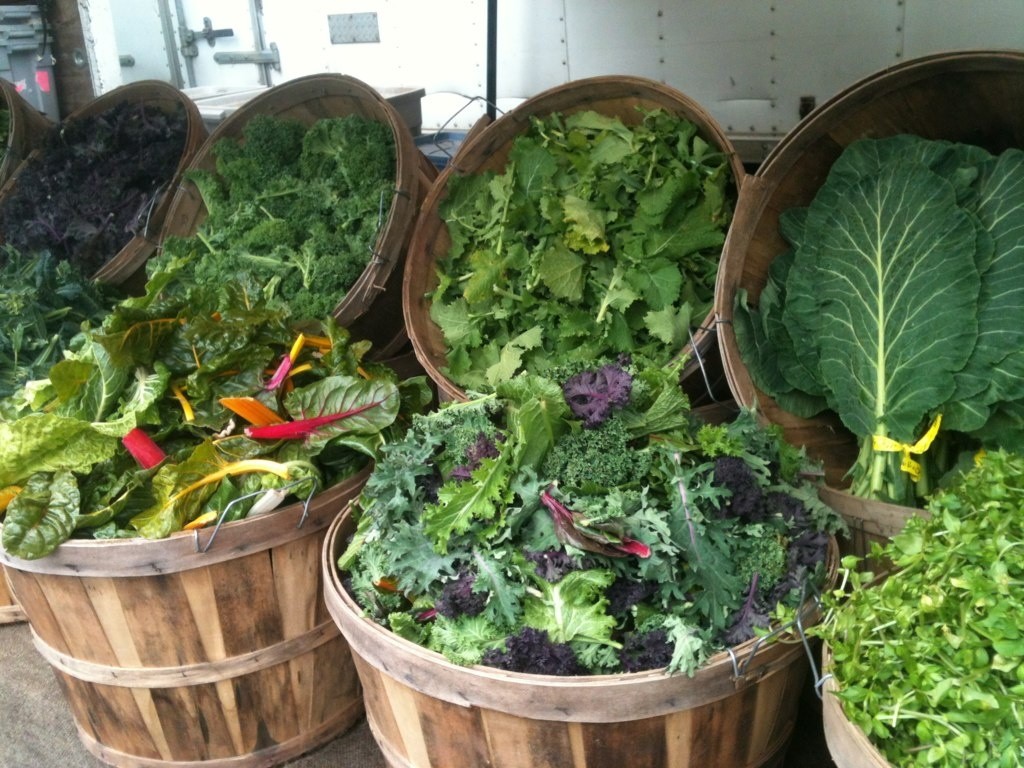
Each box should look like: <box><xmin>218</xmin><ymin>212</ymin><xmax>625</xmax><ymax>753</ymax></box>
<box><xmin>3</xmin><ymin>80</ymin><xmax>209</xmax><ymax>298</ymax></box>
<box><xmin>157</xmin><ymin>70</ymin><xmax>438</xmax><ymax>359</ymax></box>
<box><xmin>0</xmin><ymin>77</ymin><xmax>55</xmax><ymax>186</ymax></box>
<box><xmin>811</xmin><ymin>479</ymin><xmax>916</xmax><ymax>555</ymax></box>
<box><xmin>405</xmin><ymin>75</ymin><xmax>748</xmax><ymax>419</ymax></box>
<box><xmin>318</xmin><ymin>491</ymin><xmax>840</xmax><ymax>767</ymax></box>
<box><xmin>0</xmin><ymin>564</ymin><xmax>27</xmax><ymax>632</ymax></box>
<box><xmin>717</xmin><ymin>48</ymin><xmax>1022</xmax><ymax>511</ymax></box>
<box><xmin>1</xmin><ymin>467</ymin><xmax>367</xmax><ymax>767</ymax></box>
<box><xmin>813</xmin><ymin>635</ymin><xmax>903</xmax><ymax>768</ymax></box>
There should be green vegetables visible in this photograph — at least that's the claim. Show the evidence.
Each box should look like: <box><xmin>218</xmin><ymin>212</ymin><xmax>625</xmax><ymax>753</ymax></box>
<box><xmin>0</xmin><ymin>99</ymin><xmax>1024</xmax><ymax>768</ymax></box>
